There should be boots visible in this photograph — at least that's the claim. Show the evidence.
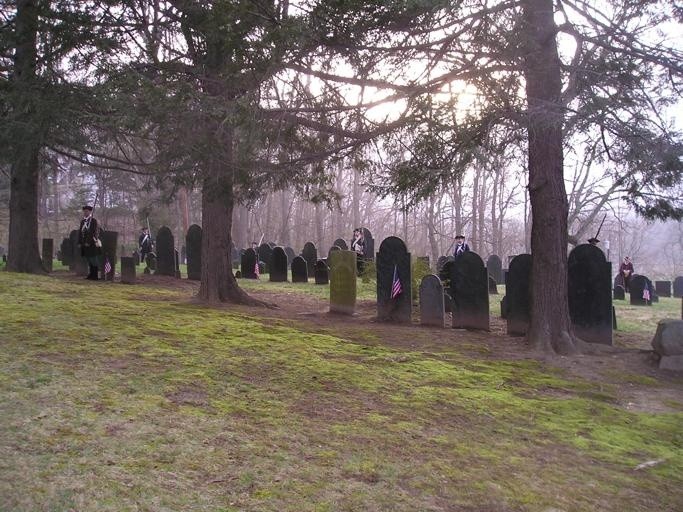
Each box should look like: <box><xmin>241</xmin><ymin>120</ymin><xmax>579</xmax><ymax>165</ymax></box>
<box><xmin>86</xmin><ymin>266</ymin><xmax>99</xmax><ymax>281</ymax></box>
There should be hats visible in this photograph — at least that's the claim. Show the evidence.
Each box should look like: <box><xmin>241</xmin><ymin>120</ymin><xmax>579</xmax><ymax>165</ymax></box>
<box><xmin>352</xmin><ymin>228</ymin><xmax>363</xmax><ymax>233</ymax></box>
<box><xmin>82</xmin><ymin>206</ymin><xmax>92</xmax><ymax>210</ymax></box>
<box><xmin>454</xmin><ymin>234</ymin><xmax>464</xmax><ymax>239</ymax></box>
<box><xmin>587</xmin><ymin>238</ymin><xmax>600</xmax><ymax>242</ymax></box>
<box><xmin>141</xmin><ymin>226</ymin><xmax>148</xmax><ymax>229</ymax></box>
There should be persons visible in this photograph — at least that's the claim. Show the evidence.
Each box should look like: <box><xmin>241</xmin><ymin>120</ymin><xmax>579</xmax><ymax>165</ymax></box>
<box><xmin>452</xmin><ymin>235</ymin><xmax>470</xmax><ymax>259</ymax></box>
<box><xmin>75</xmin><ymin>205</ymin><xmax>99</xmax><ymax>281</ymax></box>
<box><xmin>619</xmin><ymin>256</ymin><xmax>633</xmax><ymax>294</ymax></box>
<box><xmin>350</xmin><ymin>229</ymin><xmax>367</xmax><ymax>277</ymax></box>
<box><xmin>137</xmin><ymin>227</ymin><xmax>154</xmax><ymax>263</ymax></box>
<box><xmin>251</xmin><ymin>242</ymin><xmax>261</xmax><ymax>280</ymax></box>
